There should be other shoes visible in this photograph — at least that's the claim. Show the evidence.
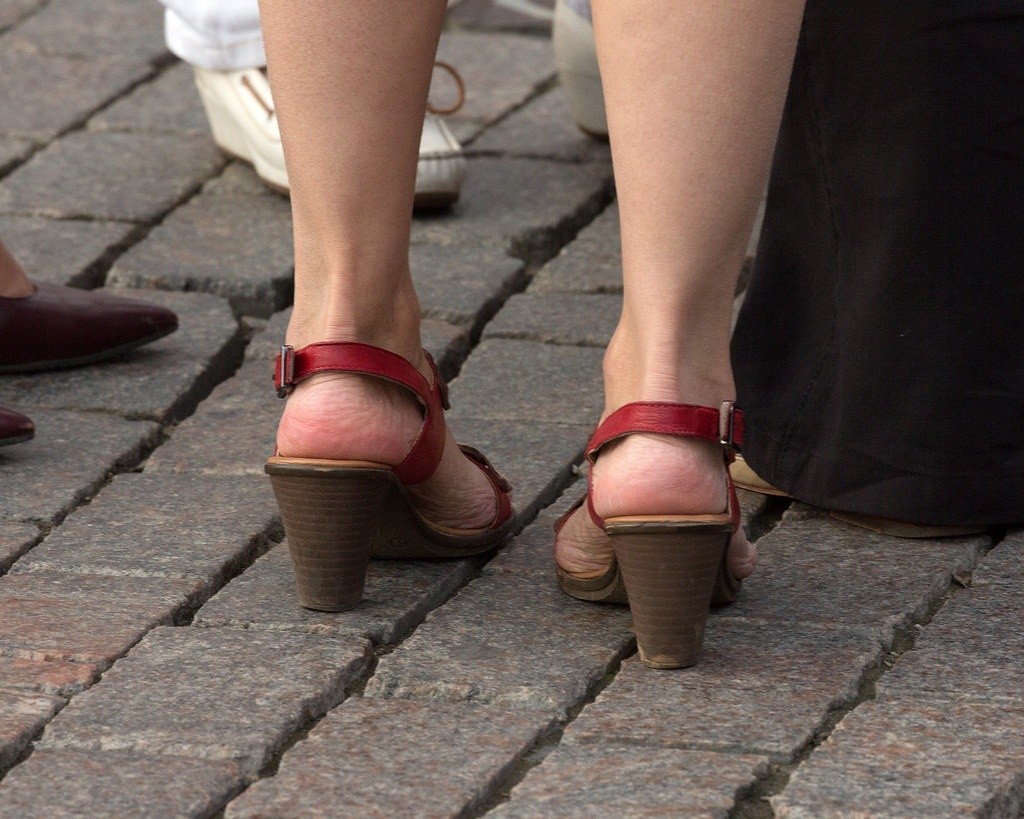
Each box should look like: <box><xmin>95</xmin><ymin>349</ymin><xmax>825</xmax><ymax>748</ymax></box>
<box><xmin>193</xmin><ymin>38</ymin><xmax>468</xmax><ymax>210</ymax></box>
<box><xmin>0</xmin><ymin>406</ymin><xmax>36</xmax><ymax>446</ymax></box>
<box><xmin>0</xmin><ymin>280</ymin><xmax>180</xmax><ymax>376</ymax></box>
<box><xmin>554</xmin><ymin>0</ymin><xmax>613</xmax><ymax>136</ymax></box>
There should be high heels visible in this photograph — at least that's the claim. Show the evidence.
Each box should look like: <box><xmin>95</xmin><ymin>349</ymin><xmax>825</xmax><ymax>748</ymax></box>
<box><xmin>262</xmin><ymin>339</ymin><xmax>519</xmax><ymax>613</ymax></box>
<box><xmin>553</xmin><ymin>398</ymin><xmax>742</xmax><ymax>670</ymax></box>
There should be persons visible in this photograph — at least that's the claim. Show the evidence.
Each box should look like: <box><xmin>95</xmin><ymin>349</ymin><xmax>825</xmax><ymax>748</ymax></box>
<box><xmin>161</xmin><ymin>0</ymin><xmax>611</xmax><ymax>209</ymax></box>
<box><xmin>733</xmin><ymin>0</ymin><xmax>1024</xmax><ymax>540</ymax></box>
<box><xmin>1</xmin><ymin>239</ymin><xmax>180</xmax><ymax>446</ymax></box>
<box><xmin>261</xmin><ymin>0</ymin><xmax>807</xmax><ymax>668</ymax></box>
